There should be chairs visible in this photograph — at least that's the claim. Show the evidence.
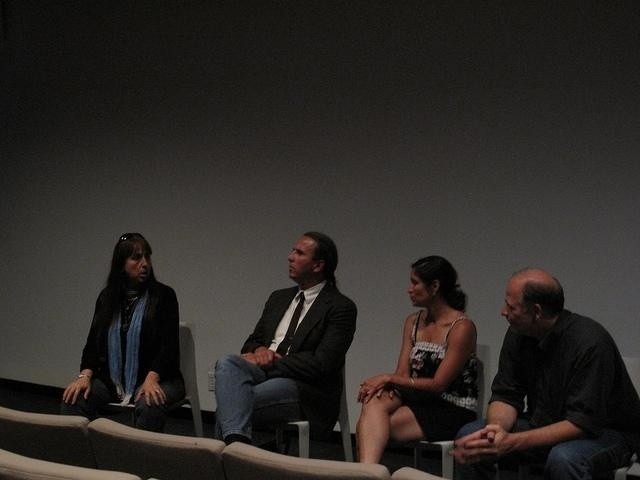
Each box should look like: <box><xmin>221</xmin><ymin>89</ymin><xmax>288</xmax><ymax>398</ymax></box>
<box><xmin>391</xmin><ymin>467</ymin><xmax>445</xmax><ymax>480</ymax></box>
<box><xmin>614</xmin><ymin>453</ymin><xmax>640</xmax><ymax>480</ymax></box>
<box><xmin>108</xmin><ymin>323</ymin><xmax>203</xmax><ymax>436</ymax></box>
<box><xmin>0</xmin><ymin>449</ymin><xmax>141</xmax><ymax>480</ymax></box>
<box><xmin>279</xmin><ymin>368</ymin><xmax>353</xmax><ymax>462</ymax></box>
<box><xmin>87</xmin><ymin>418</ymin><xmax>226</xmax><ymax>480</ymax></box>
<box><xmin>0</xmin><ymin>407</ymin><xmax>96</xmax><ymax>468</ymax></box>
<box><xmin>414</xmin><ymin>355</ymin><xmax>491</xmax><ymax>480</ymax></box>
<box><xmin>221</xmin><ymin>442</ymin><xmax>391</xmax><ymax>480</ymax></box>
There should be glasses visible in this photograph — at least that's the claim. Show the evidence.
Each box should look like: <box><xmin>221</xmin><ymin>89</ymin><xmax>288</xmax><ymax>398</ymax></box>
<box><xmin>119</xmin><ymin>233</ymin><xmax>144</xmax><ymax>241</ymax></box>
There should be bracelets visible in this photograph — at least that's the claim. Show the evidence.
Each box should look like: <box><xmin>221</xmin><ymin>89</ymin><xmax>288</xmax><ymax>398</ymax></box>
<box><xmin>74</xmin><ymin>373</ymin><xmax>89</xmax><ymax>379</ymax></box>
<box><xmin>410</xmin><ymin>376</ymin><xmax>416</xmax><ymax>392</ymax></box>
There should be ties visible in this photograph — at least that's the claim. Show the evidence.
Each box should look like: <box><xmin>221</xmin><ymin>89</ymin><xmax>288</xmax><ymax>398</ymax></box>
<box><xmin>276</xmin><ymin>292</ymin><xmax>305</xmax><ymax>354</ymax></box>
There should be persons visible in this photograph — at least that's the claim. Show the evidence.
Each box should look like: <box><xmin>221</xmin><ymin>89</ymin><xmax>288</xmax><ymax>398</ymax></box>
<box><xmin>61</xmin><ymin>232</ymin><xmax>186</xmax><ymax>433</ymax></box>
<box><xmin>356</xmin><ymin>255</ymin><xmax>479</xmax><ymax>463</ymax></box>
<box><xmin>449</xmin><ymin>268</ymin><xmax>640</xmax><ymax>480</ymax></box>
<box><xmin>214</xmin><ymin>232</ymin><xmax>358</xmax><ymax>445</ymax></box>
